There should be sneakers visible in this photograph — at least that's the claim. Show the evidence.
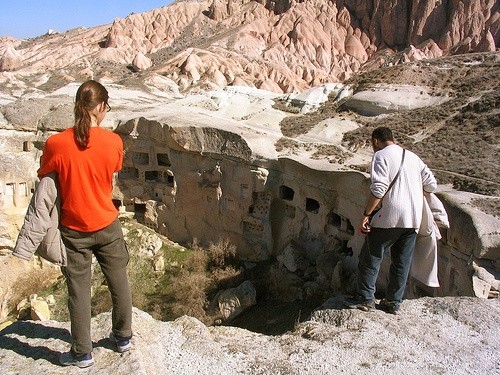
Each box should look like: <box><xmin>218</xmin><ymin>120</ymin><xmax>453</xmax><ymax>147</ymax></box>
<box><xmin>109</xmin><ymin>333</ymin><xmax>132</xmax><ymax>352</ymax></box>
<box><xmin>344</xmin><ymin>296</ymin><xmax>375</xmax><ymax>312</ymax></box>
<box><xmin>375</xmin><ymin>299</ymin><xmax>399</xmax><ymax>313</ymax></box>
<box><xmin>59</xmin><ymin>347</ymin><xmax>95</xmax><ymax>367</ymax></box>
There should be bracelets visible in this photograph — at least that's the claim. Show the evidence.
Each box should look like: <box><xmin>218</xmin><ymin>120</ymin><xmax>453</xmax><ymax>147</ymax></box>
<box><xmin>363</xmin><ymin>210</ymin><xmax>371</xmax><ymax>217</ymax></box>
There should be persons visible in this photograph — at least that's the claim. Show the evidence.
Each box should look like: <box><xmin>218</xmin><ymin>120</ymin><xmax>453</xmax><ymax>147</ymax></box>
<box><xmin>357</xmin><ymin>126</ymin><xmax>437</xmax><ymax>313</ymax></box>
<box><xmin>36</xmin><ymin>80</ymin><xmax>133</xmax><ymax>369</ymax></box>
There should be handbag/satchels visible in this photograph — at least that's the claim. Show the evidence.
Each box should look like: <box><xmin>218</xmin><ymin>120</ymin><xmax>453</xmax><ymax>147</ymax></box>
<box><xmin>360</xmin><ymin>208</ymin><xmax>382</xmax><ymax>234</ymax></box>
